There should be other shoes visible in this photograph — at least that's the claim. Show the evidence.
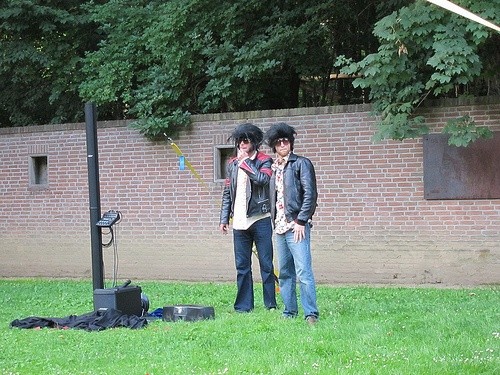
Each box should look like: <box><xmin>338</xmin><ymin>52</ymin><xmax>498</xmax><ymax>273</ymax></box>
<box><xmin>306</xmin><ymin>316</ymin><xmax>316</xmax><ymax>324</ymax></box>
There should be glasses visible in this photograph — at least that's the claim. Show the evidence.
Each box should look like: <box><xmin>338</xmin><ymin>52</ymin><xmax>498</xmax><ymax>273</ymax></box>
<box><xmin>238</xmin><ymin>138</ymin><xmax>252</xmax><ymax>144</ymax></box>
<box><xmin>275</xmin><ymin>140</ymin><xmax>291</xmax><ymax>146</ymax></box>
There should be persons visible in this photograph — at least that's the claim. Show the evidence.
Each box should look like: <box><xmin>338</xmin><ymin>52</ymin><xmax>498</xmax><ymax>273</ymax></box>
<box><xmin>267</xmin><ymin>122</ymin><xmax>319</xmax><ymax>325</ymax></box>
<box><xmin>219</xmin><ymin>123</ymin><xmax>280</xmax><ymax>313</ymax></box>
